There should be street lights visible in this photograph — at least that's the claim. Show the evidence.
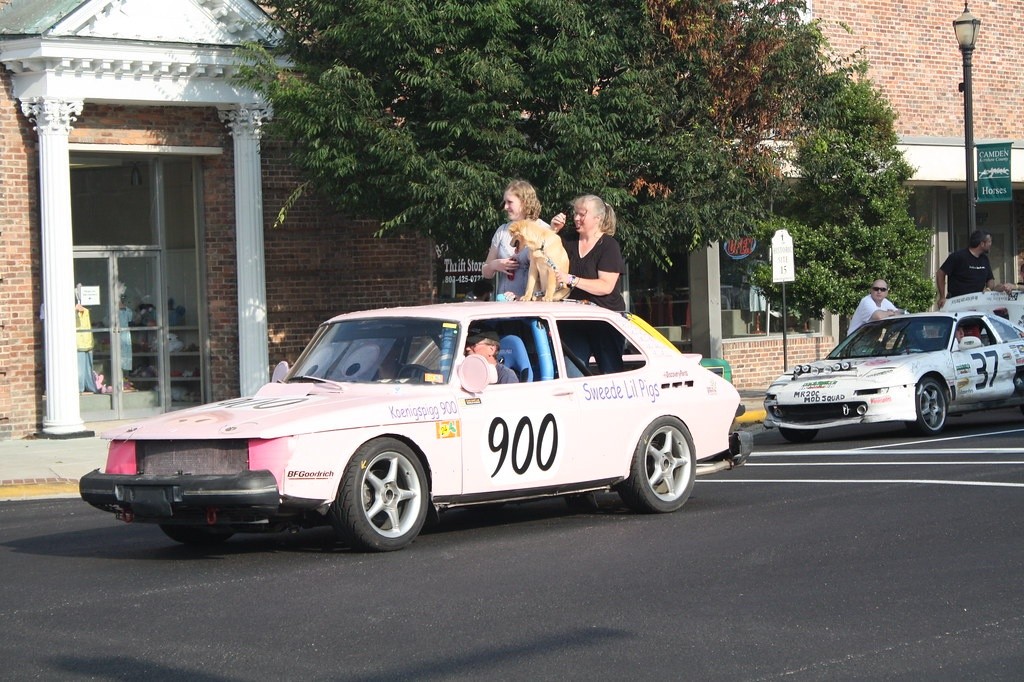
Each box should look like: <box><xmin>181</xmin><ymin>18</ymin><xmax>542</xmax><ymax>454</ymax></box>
<box><xmin>951</xmin><ymin>1</ymin><xmax>985</xmax><ymax>242</ymax></box>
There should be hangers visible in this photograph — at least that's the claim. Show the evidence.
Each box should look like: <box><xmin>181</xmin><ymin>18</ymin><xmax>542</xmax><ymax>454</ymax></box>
<box><xmin>118</xmin><ymin>299</ymin><xmax>133</xmax><ymax>315</ymax></box>
<box><xmin>73</xmin><ymin>291</ymin><xmax>91</xmax><ymax>316</ymax></box>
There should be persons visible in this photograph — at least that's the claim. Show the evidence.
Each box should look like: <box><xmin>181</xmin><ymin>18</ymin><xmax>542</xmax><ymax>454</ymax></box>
<box><xmin>955</xmin><ymin>322</ymin><xmax>966</xmax><ymax>343</ymax></box>
<box><xmin>937</xmin><ymin>228</ymin><xmax>996</xmax><ymax>310</ymax></box>
<box><xmin>464</xmin><ymin>325</ymin><xmax>519</xmax><ymax>384</ymax></box>
<box><xmin>76</xmin><ymin>298</ymin><xmax>98</xmax><ymax>395</ymax></box>
<box><xmin>481</xmin><ymin>177</ymin><xmax>551</xmax><ymax>300</ymax></box>
<box><xmin>118</xmin><ymin>293</ymin><xmax>133</xmax><ymax>371</ymax></box>
<box><xmin>549</xmin><ymin>195</ymin><xmax>626</xmax><ymax>378</ymax></box>
<box><xmin>1004</xmin><ymin>263</ymin><xmax>1024</xmax><ymax>295</ymax></box>
<box><xmin>843</xmin><ymin>278</ymin><xmax>903</xmax><ymax>356</ymax></box>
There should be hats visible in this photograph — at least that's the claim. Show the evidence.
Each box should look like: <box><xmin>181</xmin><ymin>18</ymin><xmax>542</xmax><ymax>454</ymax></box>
<box><xmin>467</xmin><ymin>324</ymin><xmax>500</xmax><ymax>343</ymax></box>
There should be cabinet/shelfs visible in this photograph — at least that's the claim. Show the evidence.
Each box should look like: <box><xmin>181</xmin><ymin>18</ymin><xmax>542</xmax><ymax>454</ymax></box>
<box><xmin>91</xmin><ymin>328</ymin><xmax>202</xmax><ymax>406</ymax></box>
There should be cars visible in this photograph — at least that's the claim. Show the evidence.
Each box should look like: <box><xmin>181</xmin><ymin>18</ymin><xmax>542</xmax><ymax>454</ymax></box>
<box><xmin>80</xmin><ymin>296</ymin><xmax>740</xmax><ymax>552</ymax></box>
<box><xmin>761</xmin><ymin>311</ymin><xmax>1024</xmax><ymax>443</ymax></box>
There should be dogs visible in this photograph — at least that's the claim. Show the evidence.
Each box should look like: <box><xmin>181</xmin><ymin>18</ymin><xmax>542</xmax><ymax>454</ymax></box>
<box><xmin>508</xmin><ymin>218</ymin><xmax>572</xmax><ymax>302</ymax></box>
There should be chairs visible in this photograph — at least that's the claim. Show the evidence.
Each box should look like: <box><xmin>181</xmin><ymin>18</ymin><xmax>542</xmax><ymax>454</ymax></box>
<box><xmin>496</xmin><ymin>334</ymin><xmax>535</xmax><ymax>383</ymax></box>
<box><xmin>907</xmin><ymin>325</ymin><xmax>995</xmax><ymax>354</ymax></box>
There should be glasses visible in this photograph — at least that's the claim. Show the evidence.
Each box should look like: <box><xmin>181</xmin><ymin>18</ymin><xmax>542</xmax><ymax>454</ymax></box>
<box><xmin>563</xmin><ymin>206</ymin><xmax>588</xmax><ymax>230</ymax></box>
<box><xmin>470</xmin><ymin>342</ymin><xmax>492</xmax><ymax>348</ymax></box>
<box><xmin>871</xmin><ymin>286</ymin><xmax>887</xmax><ymax>292</ymax></box>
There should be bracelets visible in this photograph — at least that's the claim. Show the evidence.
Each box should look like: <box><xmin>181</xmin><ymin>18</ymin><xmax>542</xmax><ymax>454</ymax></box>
<box><xmin>570</xmin><ymin>275</ymin><xmax>581</xmax><ymax>287</ymax></box>
